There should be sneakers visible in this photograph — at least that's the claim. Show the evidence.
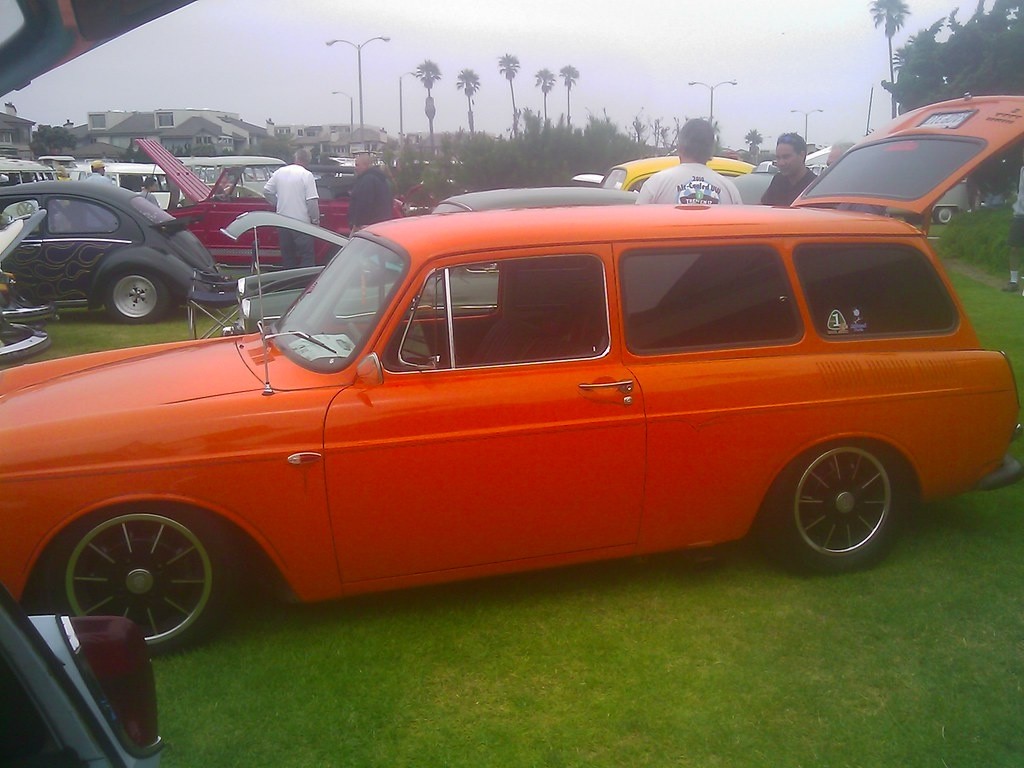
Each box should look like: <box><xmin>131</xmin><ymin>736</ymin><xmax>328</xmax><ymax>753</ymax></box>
<box><xmin>1002</xmin><ymin>283</ymin><xmax>1019</xmax><ymax>292</ymax></box>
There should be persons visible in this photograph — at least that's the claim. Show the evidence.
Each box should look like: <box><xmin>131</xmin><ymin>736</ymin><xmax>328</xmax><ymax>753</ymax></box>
<box><xmin>634</xmin><ymin>119</ymin><xmax>744</xmax><ymax>205</ymax></box>
<box><xmin>141</xmin><ymin>177</ymin><xmax>160</xmax><ymax>206</ymax></box>
<box><xmin>83</xmin><ymin>160</ymin><xmax>113</xmax><ymax>184</ymax></box>
<box><xmin>349</xmin><ymin>153</ymin><xmax>393</xmax><ymax>231</ymax></box>
<box><xmin>262</xmin><ymin>148</ymin><xmax>319</xmax><ymax>269</ymax></box>
<box><xmin>1002</xmin><ymin>166</ymin><xmax>1024</xmax><ymax>296</ymax></box>
<box><xmin>56</xmin><ymin>162</ymin><xmax>72</xmax><ymax>181</ymax></box>
<box><xmin>761</xmin><ymin>132</ymin><xmax>888</xmax><ymax>216</ymax></box>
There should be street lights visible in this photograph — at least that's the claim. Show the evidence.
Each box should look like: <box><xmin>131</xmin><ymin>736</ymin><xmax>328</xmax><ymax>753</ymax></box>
<box><xmin>333</xmin><ymin>91</ymin><xmax>354</xmax><ymax>132</ymax></box>
<box><xmin>400</xmin><ymin>72</ymin><xmax>418</xmax><ymax>139</ymax></box>
<box><xmin>325</xmin><ymin>35</ymin><xmax>391</xmax><ymax>150</ymax></box>
<box><xmin>790</xmin><ymin>109</ymin><xmax>824</xmax><ymax>144</ymax></box>
<box><xmin>689</xmin><ymin>80</ymin><xmax>738</xmax><ymax>125</ymax></box>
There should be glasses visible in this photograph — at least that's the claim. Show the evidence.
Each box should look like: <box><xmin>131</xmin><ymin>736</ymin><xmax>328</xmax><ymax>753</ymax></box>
<box><xmin>778</xmin><ymin>136</ymin><xmax>803</xmax><ymax>145</ymax></box>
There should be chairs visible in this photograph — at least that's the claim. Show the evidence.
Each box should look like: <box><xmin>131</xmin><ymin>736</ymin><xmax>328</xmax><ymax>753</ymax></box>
<box><xmin>186</xmin><ymin>239</ymin><xmax>260</xmax><ymax>340</ymax></box>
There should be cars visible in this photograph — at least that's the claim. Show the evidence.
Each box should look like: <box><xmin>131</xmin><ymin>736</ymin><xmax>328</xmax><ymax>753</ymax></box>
<box><xmin>1</xmin><ymin>92</ymin><xmax>1024</xmax><ymax>656</ymax></box>
<box><xmin>0</xmin><ymin>1</ymin><xmax>200</xmax><ymax>768</ymax></box>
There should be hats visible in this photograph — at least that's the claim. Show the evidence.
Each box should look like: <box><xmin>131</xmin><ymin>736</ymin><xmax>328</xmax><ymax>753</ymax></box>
<box><xmin>91</xmin><ymin>160</ymin><xmax>109</xmax><ymax>168</ymax></box>
